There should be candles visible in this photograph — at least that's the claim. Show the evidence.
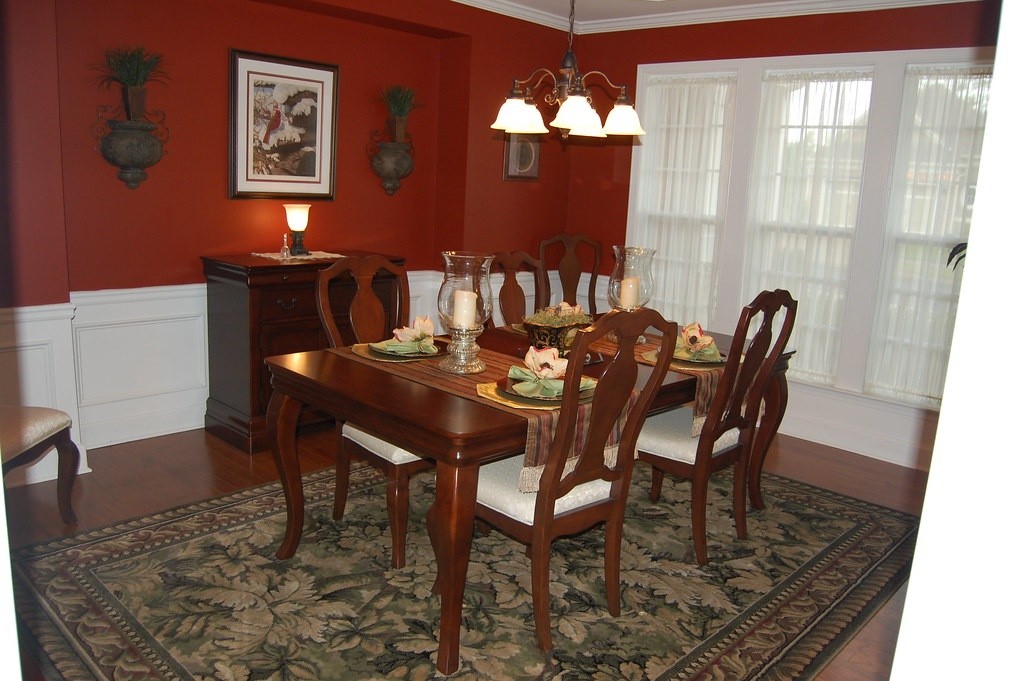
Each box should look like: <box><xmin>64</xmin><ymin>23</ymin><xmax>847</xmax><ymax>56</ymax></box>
<box><xmin>455</xmin><ymin>291</ymin><xmax>478</xmax><ymax>330</ymax></box>
<box><xmin>619</xmin><ymin>278</ymin><xmax>640</xmax><ymax>309</ymax></box>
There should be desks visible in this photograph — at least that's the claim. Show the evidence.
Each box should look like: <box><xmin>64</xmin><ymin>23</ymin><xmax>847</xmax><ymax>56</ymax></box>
<box><xmin>263</xmin><ymin>313</ymin><xmax>773</xmax><ymax>675</ymax></box>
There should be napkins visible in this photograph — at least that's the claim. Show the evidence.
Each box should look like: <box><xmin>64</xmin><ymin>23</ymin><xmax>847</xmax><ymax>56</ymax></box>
<box><xmin>383</xmin><ymin>338</ymin><xmax>439</xmax><ymax>354</ymax></box>
<box><xmin>671</xmin><ymin>337</ymin><xmax>722</xmax><ymax>361</ymax></box>
<box><xmin>507</xmin><ymin>365</ymin><xmax>591</xmax><ymax>399</ymax></box>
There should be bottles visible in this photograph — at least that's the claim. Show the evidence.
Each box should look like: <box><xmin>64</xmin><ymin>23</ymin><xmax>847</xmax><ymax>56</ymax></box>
<box><xmin>281</xmin><ymin>234</ymin><xmax>290</xmax><ymax>259</ymax></box>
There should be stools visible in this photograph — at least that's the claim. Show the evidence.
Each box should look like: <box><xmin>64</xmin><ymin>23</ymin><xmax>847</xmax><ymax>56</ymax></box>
<box><xmin>1</xmin><ymin>402</ymin><xmax>81</xmax><ymax>529</ymax></box>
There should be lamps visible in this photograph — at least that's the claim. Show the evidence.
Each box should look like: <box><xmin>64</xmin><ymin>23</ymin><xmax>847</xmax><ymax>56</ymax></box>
<box><xmin>489</xmin><ymin>0</ymin><xmax>646</xmax><ymax>139</ymax></box>
<box><xmin>285</xmin><ymin>205</ymin><xmax>312</xmax><ymax>256</ymax></box>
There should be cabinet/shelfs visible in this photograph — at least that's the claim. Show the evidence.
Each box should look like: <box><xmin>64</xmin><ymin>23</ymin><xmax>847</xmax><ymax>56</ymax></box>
<box><xmin>204</xmin><ymin>251</ymin><xmax>411</xmax><ymax>454</ymax></box>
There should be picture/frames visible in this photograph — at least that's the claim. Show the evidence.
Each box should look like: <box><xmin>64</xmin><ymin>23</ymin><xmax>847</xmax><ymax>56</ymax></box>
<box><xmin>228</xmin><ymin>49</ymin><xmax>340</xmax><ymax>199</ymax></box>
<box><xmin>502</xmin><ymin>132</ymin><xmax>539</xmax><ymax>179</ymax></box>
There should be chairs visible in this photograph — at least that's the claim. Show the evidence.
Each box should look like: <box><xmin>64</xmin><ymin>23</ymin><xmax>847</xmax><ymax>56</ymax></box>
<box><xmin>630</xmin><ymin>290</ymin><xmax>799</xmax><ymax>567</ymax></box>
<box><xmin>426</xmin><ymin>308</ymin><xmax>680</xmax><ymax>651</ymax></box>
<box><xmin>315</xmin><ymin>250</ymin><xmax>490</xmax><ymax>569</ymax></box>
<box><xmin>470</xmin><ymin>254</ymin><xmax>544</xmax><ymax>330</ymax></box>
<box><xmin>537</xmin><ymin>235</ymin><xmax>602</xmax><ymax>314</ymax></box>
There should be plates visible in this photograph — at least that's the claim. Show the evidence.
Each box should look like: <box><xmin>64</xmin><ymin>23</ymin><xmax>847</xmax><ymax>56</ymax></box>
<box><xmin>496</xmin><ymin>377</ymin><xmax>595</xmax><ymax>400</ymax></box>
<box><xmin>368</xmin><ymin>340</ymin><xmax>449</xmax><ymax>357</ymax></box>
<box><xmin>657</xmin><ymin>346</ymin><xmax>727</xmax><ymax>363</ymax></box>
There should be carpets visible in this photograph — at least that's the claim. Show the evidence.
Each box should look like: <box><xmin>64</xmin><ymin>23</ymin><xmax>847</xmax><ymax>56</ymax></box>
<box><xmin>9</xmin><ymin>464</ymin><xmax>922</xmax><ymax>681</ymax></box>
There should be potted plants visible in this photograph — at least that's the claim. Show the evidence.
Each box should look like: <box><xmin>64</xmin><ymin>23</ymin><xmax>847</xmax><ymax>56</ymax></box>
<box><xmin>90</xmin><ymin>49</ymin><xmax>170</xmax><ymax>124</ymax></box>
<box><xmin>382</xmin><ymin>88</ymin><xmax>416</xmax><ymax>141</ymax></box>
